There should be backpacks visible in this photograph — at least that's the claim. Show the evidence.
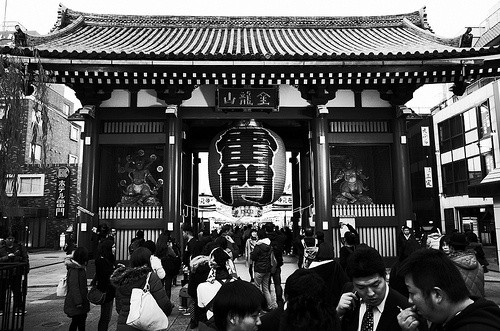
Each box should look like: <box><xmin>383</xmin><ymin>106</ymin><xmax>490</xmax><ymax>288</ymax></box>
<box><xmin>300</xmin><ymin>238</ymin><xmax>319</xmax><ymax>269</ymax></box>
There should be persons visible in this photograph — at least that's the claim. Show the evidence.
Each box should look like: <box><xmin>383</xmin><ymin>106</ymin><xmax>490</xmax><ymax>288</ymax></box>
<box><xmin>123</xmin><ymin>158</ymin><xmax>158</xmax><ymax>206</ymax></box>
<box><xmin>60</xmin><ymin>217</ymin><xmax>500</xmax><ymax>331</ymax></box>
<box><xmin>333</xmin><ymin>156</ymin><xmax>370</xmax><ymax>203</ymax></box>
<box><xmin>0</xmin><ymin>236</ymin><xmax>29</xmax><ymax>316</ymax></box>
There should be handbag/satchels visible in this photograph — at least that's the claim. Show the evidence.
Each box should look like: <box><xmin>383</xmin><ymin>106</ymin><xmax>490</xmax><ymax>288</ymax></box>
<box><xmin>57</xmin><ymin>276</ymin><xmax>68</xmax><ymax>297</ymax></box>
<box><xmin>125</xmin><ymin>287</ymin><xmax>168</xmax><ymax>331</ymax></box>
<box><xmin>86</xmin><ymin>287</ymin><xmax>106</xmax><ymax>305</ymax></box>
<box><xmin>269</xmin><ymin>246</ymin><xmax>278</xmax><ymax>274</ymax></box>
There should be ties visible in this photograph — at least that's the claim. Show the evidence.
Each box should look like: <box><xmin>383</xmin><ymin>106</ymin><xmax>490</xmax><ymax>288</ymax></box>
<box><xmin>361</xmin><ymin>304</ymin><xmax>374</xmax><ymax>331</ymax></box>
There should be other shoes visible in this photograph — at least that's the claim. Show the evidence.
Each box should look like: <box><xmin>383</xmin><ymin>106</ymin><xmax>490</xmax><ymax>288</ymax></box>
<box><xmin>182</xmin><ymin>308</ymin><xmax>191</xmax><ymax>316</ymax></box>
